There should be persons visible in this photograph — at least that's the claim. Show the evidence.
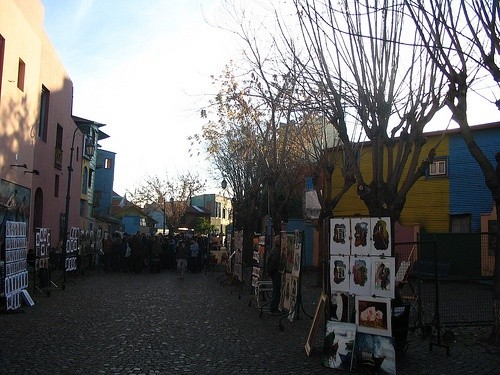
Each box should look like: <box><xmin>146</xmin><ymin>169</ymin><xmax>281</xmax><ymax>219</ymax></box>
<box><xmin>101</xmin><ymin>229</ymin><xmax>222</xmax><ymax>278</ymax></box>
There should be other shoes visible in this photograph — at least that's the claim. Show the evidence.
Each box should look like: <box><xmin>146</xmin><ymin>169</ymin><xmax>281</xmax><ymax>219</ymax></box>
<box><xmin>268</xmin><ymin>309</ymin><xmax>285</xmax><ymax>316</ymax></box>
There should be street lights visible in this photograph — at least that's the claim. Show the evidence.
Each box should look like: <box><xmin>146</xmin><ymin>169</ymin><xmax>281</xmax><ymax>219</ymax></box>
<box><xmin>60</xmin><ymin>124</ymin><xmax>96</xmax><ymax>270</ymax></box>
<box><xmin>221</xmin><ymin>178</ymin><xmax>235</xmax><ymax>235</ymax></box>
<box><xmin>163</xmin><ymin>197</ymin><xmax>173</xmax><ymax>235</ymax></box>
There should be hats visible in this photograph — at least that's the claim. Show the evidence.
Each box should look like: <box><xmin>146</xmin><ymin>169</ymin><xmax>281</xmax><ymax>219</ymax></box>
<box><xmin>179</xmin><ymin>239</ymin><xmax>184</xmax><ymax>244</ymax></box>
